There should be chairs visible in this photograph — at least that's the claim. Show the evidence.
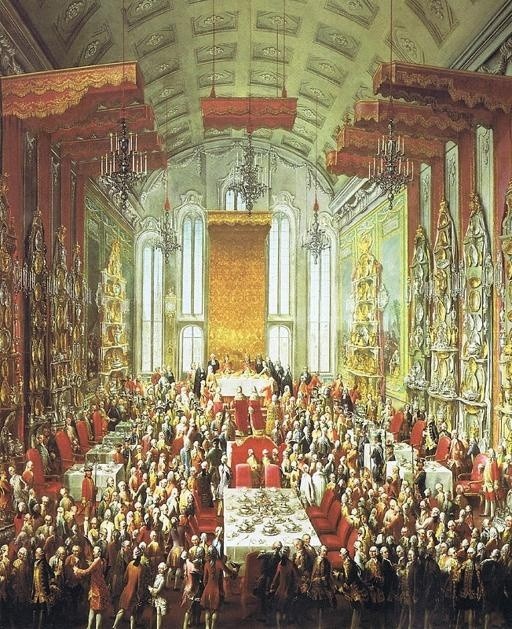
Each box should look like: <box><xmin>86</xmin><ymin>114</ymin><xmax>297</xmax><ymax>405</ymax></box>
<box><xmin>388</xmin><ymin>411</ymin><xmax>489</xmax><ymax>509</ymax></box>
<box><xmin>307</xmin><ymin>487</ymin><xmax>358</xmax><ymax>570</ymax></box>
<box><xmin>184</xmin><ymin>490</ymin><xmax>219</xmax><ymax>547</ymax></box>
<box><xmin>233</xmin><ymin>463</ymin><xmax>281</xmax><ymax>487</ymax></box>
<box><xmin>23</xmin><ymin>411</ymin><xmax>104</xmax><ymax>495</ymax></box>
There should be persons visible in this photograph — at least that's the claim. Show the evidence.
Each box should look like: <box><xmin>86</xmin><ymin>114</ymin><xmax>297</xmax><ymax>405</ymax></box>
<box><xmin>0</xmin><ymin>352</ymin><xmax>512</xmax><ymax>628</ymax></box>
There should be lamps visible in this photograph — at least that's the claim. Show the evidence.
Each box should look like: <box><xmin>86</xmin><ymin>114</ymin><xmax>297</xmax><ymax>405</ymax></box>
<box><xmin>97</xmin><ymin>0</ymin><xmax>185</xmax><ymax>266</ymax></box>
<box><xmin>300</xmin><ymin>0</ymin><xmax>417</xmax><ymax>265</ymax></box>
<box><xmin>225</xmin><ymin>0</ymin><xmax>272</xmax><ymax>216</ymax></box>
